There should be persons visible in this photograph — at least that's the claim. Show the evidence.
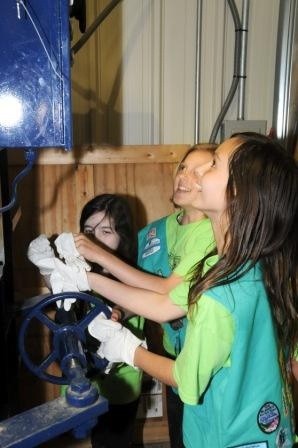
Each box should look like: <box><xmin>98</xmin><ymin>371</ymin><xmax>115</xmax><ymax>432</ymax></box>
<box><xmin>48</xmin><ymin>193</ymin><xmax>149</xmax><ymax>445</ymax></box>
<box><xmin>48</xmin><ymin>130</ymin><xmax>297</xmax><ymax>447</ymax></box>
<box><xmin>54</xmin><ymin>142</ymin><xmax>218</xmax><ymax>447</ymax></box>
